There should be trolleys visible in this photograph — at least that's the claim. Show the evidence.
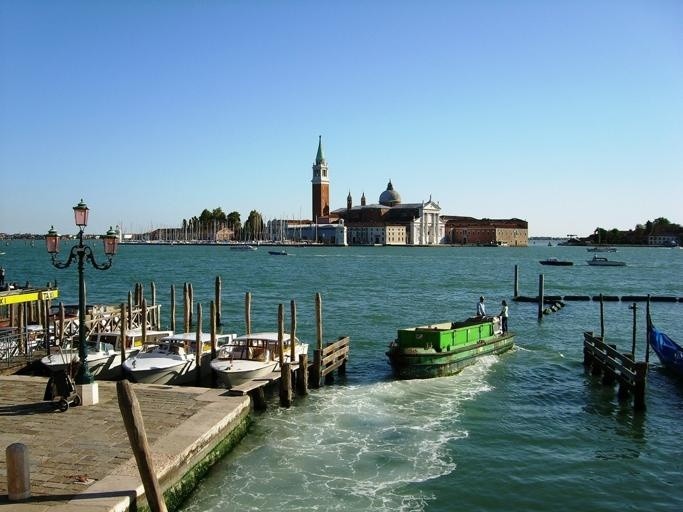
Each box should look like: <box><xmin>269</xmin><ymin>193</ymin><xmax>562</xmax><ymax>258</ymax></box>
<box><xmin>43</xmin><ymin>370</ymin><xmax>81</xmax><ymax>412</ymax></box>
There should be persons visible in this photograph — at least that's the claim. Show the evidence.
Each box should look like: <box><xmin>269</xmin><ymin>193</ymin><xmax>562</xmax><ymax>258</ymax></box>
<box><xmin>477</xmin><ymin>296</ymin><xmax>485</xmax><ymax>316</ymax></box>
<box><xmin>9</xmin><ymin>280</ymin><xmax>31</xmax><ymax>289</ymax></box>
<box><xmin>0</xmin><ymin>266</ymin><xmax>5</xmax><ymax>286</ymax></box>
<box><xmin>499</xmin><ymin>300</ymin><xmax>508</xmax><ymax>336</ymax></box>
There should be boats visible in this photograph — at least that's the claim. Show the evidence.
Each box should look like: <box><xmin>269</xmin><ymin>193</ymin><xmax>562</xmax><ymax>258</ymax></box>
<box><xmin>585</xmin><ymin>257</ymin><xmax>626</xmax><ymax>266</ymax></box>
<box><xmin>119</xmin><ymin>218</ymin><xmax>324</xmax><ymax>246</ymax></box>
<box><xmin>539</xmin><ymin>258</ymin><xmax>573</xmax><ymax>266</ymax></box>
<box><xmin>385</xmin><ymin>315</ymin><xmax>515</xmax><ymax>380</ymax></box>
<box><xmin>229</xmin><ymin>245</ymin><xmax>258</xmax><ymax>251</ymax></box>
<box><xmin>268</xmin><ymin>251</ymin><xmax>287</xmax><ymax>255</ymax></box>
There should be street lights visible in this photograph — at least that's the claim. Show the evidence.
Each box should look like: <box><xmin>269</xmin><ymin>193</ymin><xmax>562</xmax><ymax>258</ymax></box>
<box><xmin>45</xmin><ymin>198</ymin><xmax>118</xmax><ymax>384</ymax></box>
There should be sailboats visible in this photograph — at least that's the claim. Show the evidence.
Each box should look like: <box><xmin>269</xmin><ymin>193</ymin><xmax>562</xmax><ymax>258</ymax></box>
<box><xmin>586</xmin><ymin>227</ymin><xmax>617</xmax><ymax>252</ymax></box>
